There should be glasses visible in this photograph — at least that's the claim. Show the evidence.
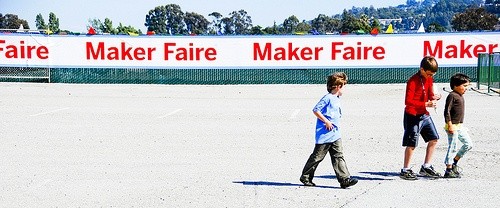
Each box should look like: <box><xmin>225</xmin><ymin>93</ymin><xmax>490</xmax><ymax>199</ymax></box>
<box><xmin>423</xmin><ymin>69</ymin><xmax>436</xmax><ymax>76</ymax></box>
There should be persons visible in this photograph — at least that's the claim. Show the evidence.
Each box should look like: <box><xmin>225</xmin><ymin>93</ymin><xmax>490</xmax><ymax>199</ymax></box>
<box><xmin>400</xmin><ymin>56</ymin><xmax>442</xmax><ymax>180</ymax></box>
<box><xmin>443</xmin><ymin>73</ymin><xmax>472</xmax><ymax>178</ymax></box>
<box><xmin>299</xmin><ymin>72</ymin><xmax>358</xmax><ymax>188</ymax></box>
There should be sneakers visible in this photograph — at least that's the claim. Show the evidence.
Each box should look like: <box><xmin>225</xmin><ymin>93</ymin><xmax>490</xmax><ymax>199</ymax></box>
<box><xmin>444</xmin><ymin>165</ymin><xmax>461</xmax><ymax>178</ymax></box>
<box><xmin>300</xmin><ymin>177</ymin><xmax>316</xmax><ymax>187</ymax></box>
<box><xmin>419</xmin><ymin>165</ymin><xmax>441</xmax><ymax>178</ymax></box>
<box><xmin>400</xmin><ymin>169</ymin><xmax>418</xmax><ymax>180</ymax></box>
<box><xmin>340</xmin><ymin>178</ymin><xmax>358</xmax><ymax>189</ymax></box>
